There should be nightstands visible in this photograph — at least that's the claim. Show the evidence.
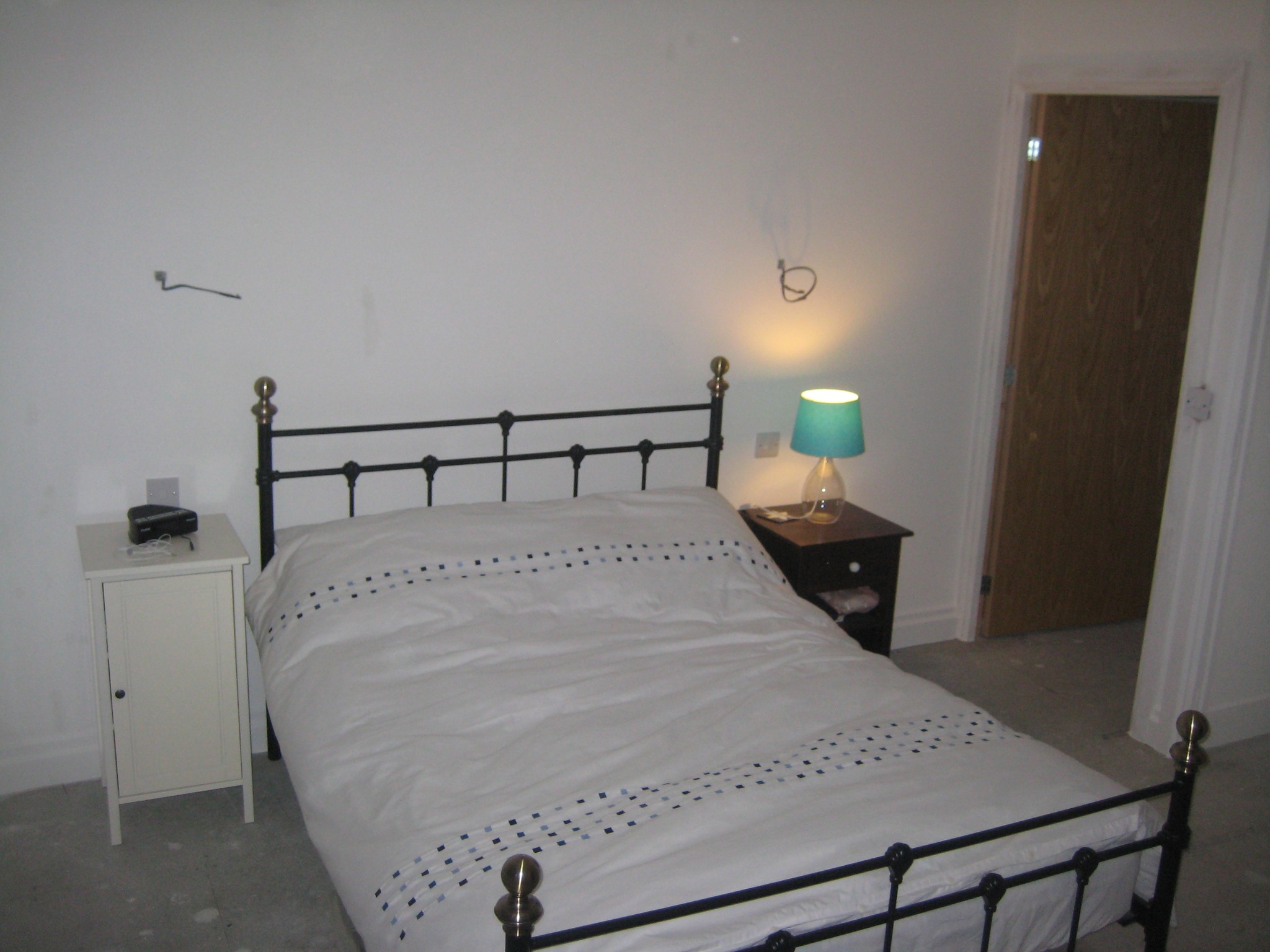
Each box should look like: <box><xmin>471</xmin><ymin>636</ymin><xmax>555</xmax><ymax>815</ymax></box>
<box><xmin>73</xmin><ymin>512</ymin><xmax>253</xmax><ymax>844</ymax></box>
<box><xmin>741</xmin><ymin>498</ymin><xmax>914</xmax><ymax>658</ymax></box>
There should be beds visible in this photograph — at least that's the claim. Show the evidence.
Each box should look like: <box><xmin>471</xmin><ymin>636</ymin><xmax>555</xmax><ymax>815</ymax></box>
<box><xmin>251</xmin><ymin>358</ymin><xmax>1208</xmax><ymax>952</ymax></box>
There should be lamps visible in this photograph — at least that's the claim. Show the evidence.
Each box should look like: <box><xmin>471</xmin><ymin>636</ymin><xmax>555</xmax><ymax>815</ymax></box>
<box><xmin>790</xmin><ymin>388</ymin><xmax>864</xmax><ymax>524</ymax></box>
